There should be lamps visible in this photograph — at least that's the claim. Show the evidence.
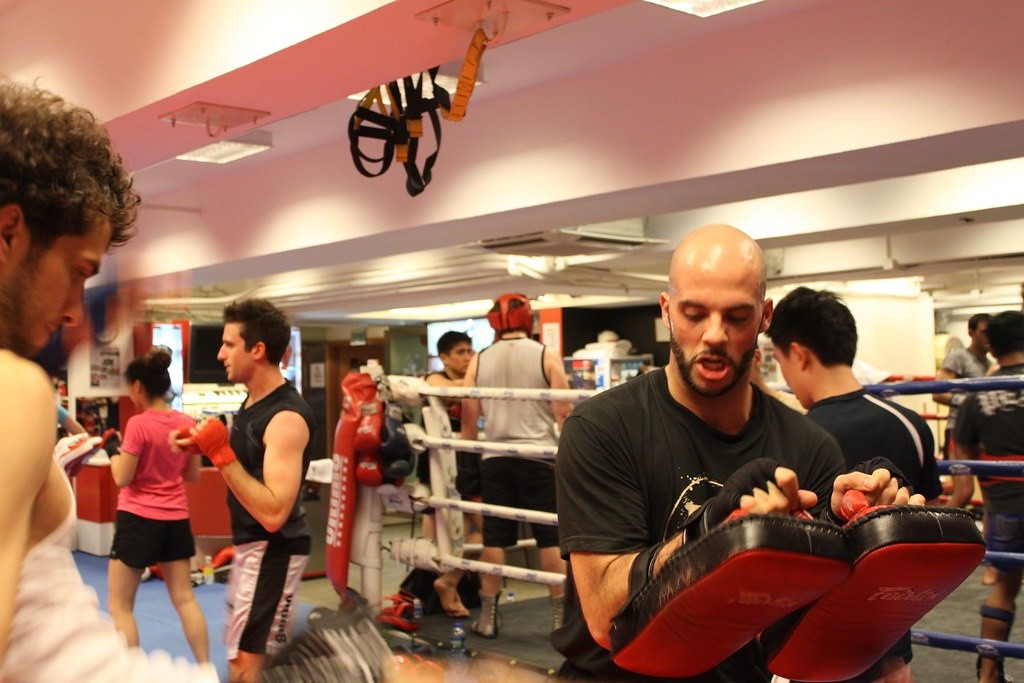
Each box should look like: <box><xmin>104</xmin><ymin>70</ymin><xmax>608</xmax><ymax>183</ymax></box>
<box><xmin>176</xmin><ymin>129</ymin><xmax>274</xmax><ymax>163</ymax></box>
<box><xmin>347</xmin><ymin>56</ymin><xmax>482</xmax><ymax>106</ymax></box>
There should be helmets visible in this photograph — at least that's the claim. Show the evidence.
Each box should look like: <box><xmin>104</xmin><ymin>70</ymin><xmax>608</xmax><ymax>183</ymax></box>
<box><xmin>487</xmin><ymin>293</ymin><xmax>535</xmax><ymax>338</ymax></box>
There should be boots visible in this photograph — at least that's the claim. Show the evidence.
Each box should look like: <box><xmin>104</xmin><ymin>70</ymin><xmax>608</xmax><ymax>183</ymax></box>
<box><xmin>549</xmin><ymin>595</ymin><xmax>566</xmax><ymax>632</ymax></box>
<box><xmin>471</xmin><ymin>593</ymin><xmax>500</xmax><ymax>637</ymax></box>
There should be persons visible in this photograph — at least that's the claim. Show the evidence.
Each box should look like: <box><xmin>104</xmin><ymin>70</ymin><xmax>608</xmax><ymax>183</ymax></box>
<box><xmin>168</xmin><ymin>297</ymin><xmax>314</xmax><ymax>683</ymax></box>
<box><xmin>553</xmin><ymin>223</ymin><xmax>927</xmax><ymax>683</ymax></box>
<box><xmin>459</xmin><ymin>293</ymin><xmax>572</xmax><ymax>638</ymax></box>
<box><xmin>767</xmin><ymin>287</ymin><xmax>945</xmax><ymax>683</ymax></box>
<box><xmin>952</xmin><ymin>310</ymin><xmax>1024</xmax><ymax>683</ymax></box>
<box><xmin>0</xmin><ymin>85</ymin><xmax>215</xmax><ymax>683</ymax></box>
<box><xmin>99</xmin><ymin>352</ymin><xmax>210</xmax><ymax>666</ymax></box>
<box><xmin>933</xmin><ymin>312</ymin><xmax>995</xmax><ymax>508</ymax></box>
<box><xmin>393</xmin><ymin>330</ymin><xmax>484</xmax><ymax>618</ymax></box>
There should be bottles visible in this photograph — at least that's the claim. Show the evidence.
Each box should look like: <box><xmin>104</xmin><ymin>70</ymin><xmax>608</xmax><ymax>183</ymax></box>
<box><xmin>506</xmin><ymin>592</ymin><xmax>515</xmax><ymax>602</ymax></box>
<box><xmin>451</xmin><ymin>623</ymin><xmax>466</xmax><ymax>660</ymax></box>
<box><xmin>412</xmin><ymin>598</ymin><xmax>423</xmax><ymax>619</ymax></box>
<box><xmin>204</xmin><ymin>555</ymin><xmax>214</xmax><ymax>586</ymax></box>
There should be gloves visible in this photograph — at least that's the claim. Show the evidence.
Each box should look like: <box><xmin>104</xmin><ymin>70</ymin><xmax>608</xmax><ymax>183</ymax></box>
<box><xmin>354</xmin><ymin>397</ymin><xmax>410</xmax><ymax>488</ymax></box>
<box><xmin>191</xmin><ymin>420</ymin><xmax>236</xmax><ymax>472</ymax></box>
